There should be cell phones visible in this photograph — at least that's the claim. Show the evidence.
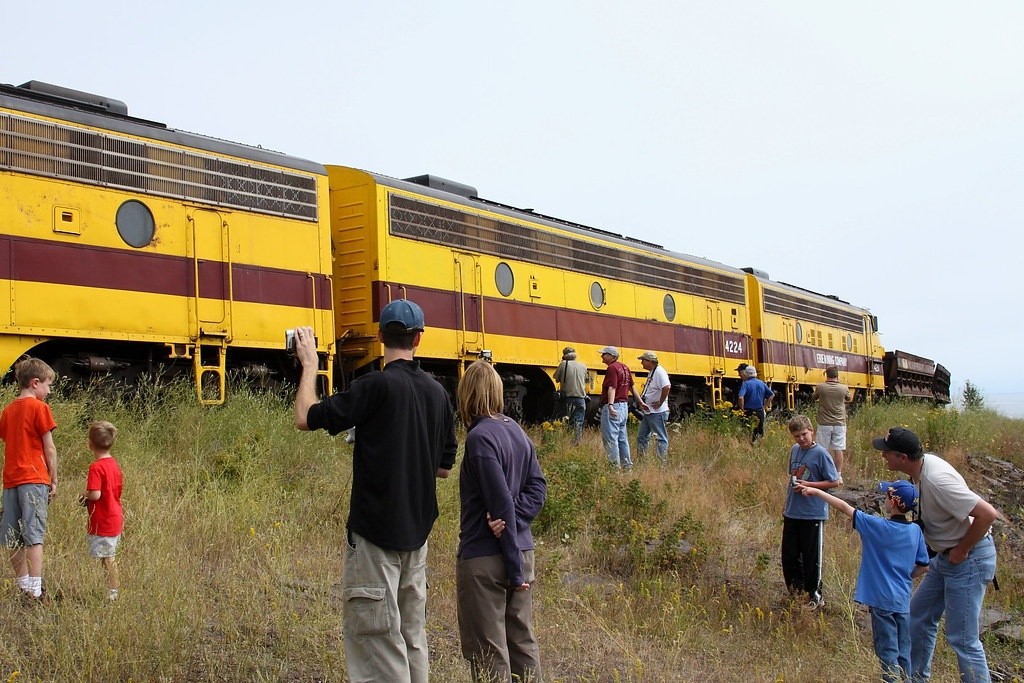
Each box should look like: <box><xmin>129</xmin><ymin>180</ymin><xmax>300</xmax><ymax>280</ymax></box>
<box><xmin>285</xmin><ymin>330</ymin><xmax>318</xmax><ymax>352</ymax></box>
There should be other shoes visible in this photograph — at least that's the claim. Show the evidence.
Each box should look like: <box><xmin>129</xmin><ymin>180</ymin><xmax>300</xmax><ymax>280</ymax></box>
<box><xmin>839</xmin><ymin>476</ymin><xmax>843</xmax><ymax>485</ymax></box>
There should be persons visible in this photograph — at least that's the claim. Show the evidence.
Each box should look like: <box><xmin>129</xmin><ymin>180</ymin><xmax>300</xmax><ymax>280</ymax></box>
<box><xmin>78</xmin><ymin>421</ymin><xmax>124</xmax><ymax>601</ymax></box>
<box><xmin>782</xmin><ymin>415</ymin><xmax>840</xmax><ymax>611</ymax></box>
<box><xmin>597</xmin><ymin>346</ymin><xmax>651</xmax><ymax>475</ymax></box>
<box><xmin>734</xmin><ymin>363</ymin><xmax>775</xmax><ymax>442</ymax></box>
<box><xmin>293</xmin><ymin>299</ymin><xmax>458</xmax><ymax>683</ymax></box>
<box><xmin>873</xmin><ymin>428</ymin><xmax>997</xmax><ymax>683</ymax></box>
<box><xmin>458</xmin><ymin>359</ymin><xmax>547</xmax><ymax>683</ymax></box>
<box><xmin>553</xmin><ymin>347</ymin><xmax>590</xmax><ymax>447</ymax></box>
<box><xmin>637</xmin><ymin>352</ymin><xmax>671</xmax><ymax>463</ymax></box>
<box><xmin>798</xmin><ymin>479</ymin><xmax>930</xmax><ymax>683</ymax></box>
<box><xmin>0</xmin><ymin>353</ymin><xmax>61</xmax><ymax>607</ymax></box>
<box><xmin>812</xmin><ymin>366</ymin><xmax>851</xmax><ymax>485</ymax></box>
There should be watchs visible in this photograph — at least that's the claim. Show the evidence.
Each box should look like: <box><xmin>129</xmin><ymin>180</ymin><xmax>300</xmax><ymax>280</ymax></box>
<box><xmin>607</xmin><ymin>403</ymin><xmax>613</xmax><ymax>407</ymax></box>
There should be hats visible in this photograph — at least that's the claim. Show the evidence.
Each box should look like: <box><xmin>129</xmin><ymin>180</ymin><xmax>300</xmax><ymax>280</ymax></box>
<box><xmin>879</xmin><ymin>480</ymin><xmax>919</xmax><ymax>510</ymax></box>
<box><xmin>826</xmin><ymin>366</ymin><xmax>838</xmax><ymax>377</ymax></box>
<box><xmin>872</xmin><ymin>428</ymin><xmax>922</xmax><ymax>453</ymax></box>
<box><xmin>379</xmin><ymin>299</ymin><xmax>424</xmax><ymax>335</ymax></box>
<box><xmin>734</xmin><ymin>363</ymin><xmax>748</xmax><ymax>370</ymax></box>
<box><xmin>744</xmin><ymin>366</ymin><xmax>757</xmax><ymax>377</ymax></box>
<box><xmin>637</xmin><ymin>352</ymin><xmax>657</xmax><ymax>361</ymax></box>
<box><xmin>563</xmin><ymin>347</ymin><xmax>576</xmax><ymax>355</ymax></box>
<box><xmin>597</xmin><ymin>346</ymin><xmax>618</xmax><ymax>357</ymax></box>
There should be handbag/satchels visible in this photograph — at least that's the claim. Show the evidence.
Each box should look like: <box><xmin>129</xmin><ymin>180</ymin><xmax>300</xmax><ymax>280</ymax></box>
<box><xmin>593</xmin><ymin>405</ymin><xmax>602</xmax><ymax>423</ymax></box>
<box><xmin>553</xmin><ymin>391</ymin><xmax>562</xmax><ymax>400</ymax></box>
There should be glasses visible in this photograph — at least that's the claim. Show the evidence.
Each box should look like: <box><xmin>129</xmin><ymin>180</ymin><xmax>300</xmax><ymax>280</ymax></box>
<box><xmin>602</xmin><ymin>352</ymin><xmax>611</xmax><ymax>356</ymax></box>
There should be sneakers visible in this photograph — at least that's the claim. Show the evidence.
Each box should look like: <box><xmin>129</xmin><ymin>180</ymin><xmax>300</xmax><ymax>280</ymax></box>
<box><xmin>14</xmin><ymin>587</ymin><xmax>54</xmax><ymax>607</ymax></box>
<box><xmin>802</xmin><ymin>596</ymin><xmax>825</xmax><ymax>611</ymax></box>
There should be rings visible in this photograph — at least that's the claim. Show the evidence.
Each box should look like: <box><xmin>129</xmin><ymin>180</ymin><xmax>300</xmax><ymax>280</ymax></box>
<box><xmin>300</xmin><ymin>334</ymin><xmax>305</xmax><ymax>337</ymax></box>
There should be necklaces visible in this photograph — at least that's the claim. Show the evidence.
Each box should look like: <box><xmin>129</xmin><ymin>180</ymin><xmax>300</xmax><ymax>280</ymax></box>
<box><xmin>792</xmin><ymin>441</ymin><xmax>813</xmax><ymax>481</ymax></box>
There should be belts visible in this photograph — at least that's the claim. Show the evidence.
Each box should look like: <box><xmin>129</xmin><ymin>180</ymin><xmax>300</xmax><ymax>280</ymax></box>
<box><xmin>601</xmin><ymin>401</ymin><xmax>623</xmax><ymax>404</ymax></box>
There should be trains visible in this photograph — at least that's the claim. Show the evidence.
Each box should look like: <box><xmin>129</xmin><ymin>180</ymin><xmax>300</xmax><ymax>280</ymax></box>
<box><xmin>0</xmin><ymin>80</ymin><xmax>951</xmax><ymax>423</ymax></box>
